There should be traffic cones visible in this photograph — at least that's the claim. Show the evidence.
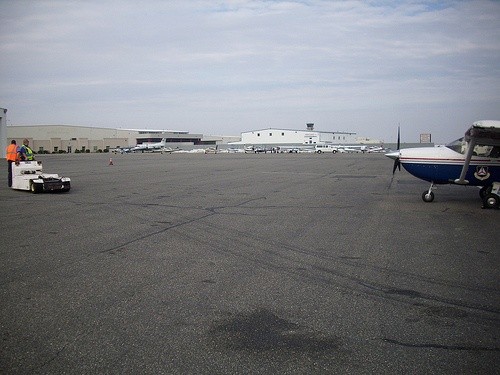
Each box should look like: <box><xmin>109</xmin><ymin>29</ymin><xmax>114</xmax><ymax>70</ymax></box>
<box><xmin>108</xmin><ymin>157</ymin><xmax>113</xmax><ymax>165</ymax></box>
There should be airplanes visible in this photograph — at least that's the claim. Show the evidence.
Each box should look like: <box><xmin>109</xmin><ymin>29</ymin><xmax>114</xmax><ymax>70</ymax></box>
<box><xmin>134</xmin><ymin>137</ymin><xmax>173</xmax><ymax>154</ymax></box>
<box><xmin>384</xmin><ymin>120</ymin><xmax>500</xmax><ymax>209</ymax></box>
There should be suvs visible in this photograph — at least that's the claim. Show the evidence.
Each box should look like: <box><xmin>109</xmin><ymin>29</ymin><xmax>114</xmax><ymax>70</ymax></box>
<box><xmin>315</xmin><ymin>144</ymin><xmax>338</xmax><ymax>154</ymax></box>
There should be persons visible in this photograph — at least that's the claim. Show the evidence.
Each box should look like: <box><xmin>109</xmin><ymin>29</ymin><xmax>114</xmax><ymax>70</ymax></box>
<box><xmin>6</xmin><ymin>140</ymin><xmax>20</xmax><ymax>188</ymax></box>
<box><xmin>20</xmin><ymin>138</ymin><xmax>36</xmax><ymax>161</ymax></box>
